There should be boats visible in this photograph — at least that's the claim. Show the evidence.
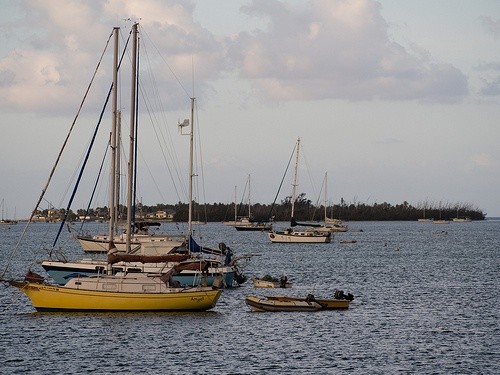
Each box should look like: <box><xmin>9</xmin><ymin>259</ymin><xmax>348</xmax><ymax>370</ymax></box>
<box><xmin>310</xmin><ymin>170</ymin><xmax>342</xmax><ymax>224</ymax></box>
<box><xmin>307</xmin><ymin>224</ymin><xmax>349</xmax><ymax>232</ymax></box>
<box><xmin>233</xmin><ymin>173</ymin><xmax>273</xmax><ymax>231</ymax></box>
<box><xmin>433</xmin><ymin>220</ymin><xmax>450</xmax><ymax>224</ymax></box>
<box><xmin>251</xmin><ymin>274</ymin><xmax>293</xmax><ymax>288</ymax></box>
<box><xmin>418</xmin><ymin>218</ymin><xmax>434</xmax><ymax>221</ymax></box>
<box><xmin>244</xmin><ymin>290</ymin><xmax>355</xmax><ymax>310</ymax></box>
<box><xmin>188</xmin><ymin>220</ymin><xmax>205</xmax><ymax>224</ymax></box>
<box><xmin>453</xmin><ymin>217</ymin><xmax>472</xmax><ymax>222</ymax></box>
<box><xmin>261</xmin><ymin>136</ymin><xmax>335</xmax><ymax>243</ymax></box>
<box><xmin>2</xmin><ymin>16</ymin><xmax>263</xmax><ymax>311</ymax></box>
<box><xmin>224</xmin><ymin>184</ymin><xmax>258</xmax><ymax>225</ymax></box>
